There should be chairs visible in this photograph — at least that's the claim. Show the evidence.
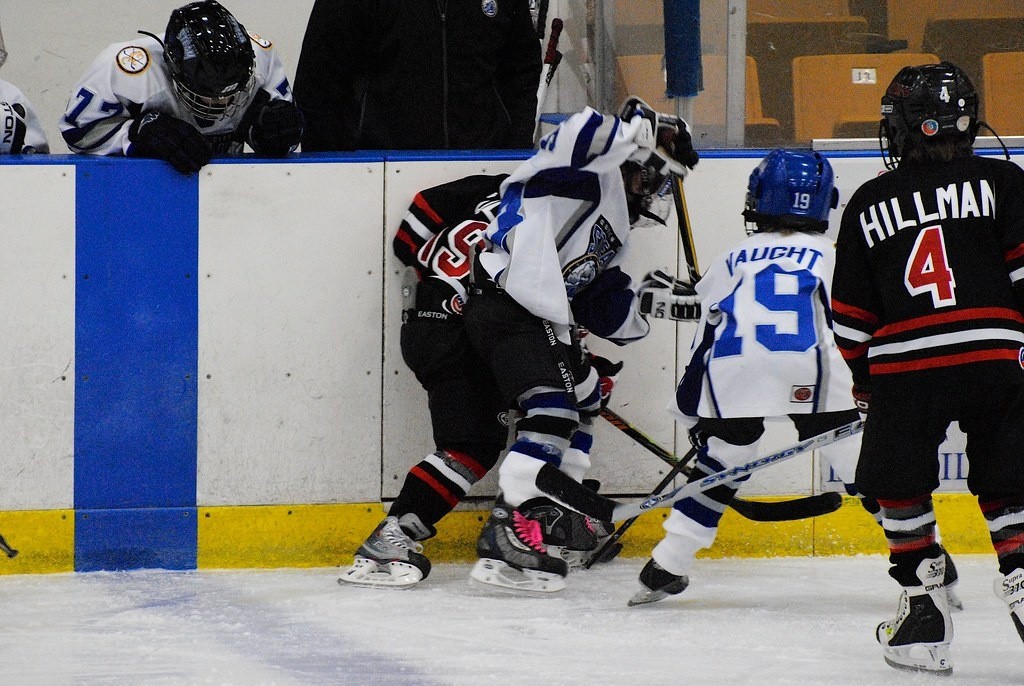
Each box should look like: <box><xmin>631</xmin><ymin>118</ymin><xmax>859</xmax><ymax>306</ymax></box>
<box><xmin>602</xmin><ymin>1</ymin><xmax>1024</xmax><ymax>150</ymax></box>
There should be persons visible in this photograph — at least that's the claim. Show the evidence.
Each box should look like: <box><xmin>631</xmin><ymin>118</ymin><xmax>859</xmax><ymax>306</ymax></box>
<box><xmin>830</xmin><ymin>60</ymin><xmax>1024</xmax><ymax>678</ymax></box>
<box><xmin>463</xmin><ymin>94</ymin><xmax>703</xmax><ymax>601</ymax></box>
<box><xmin>57</xmin><ymin>0</ymin><xmax>308</xmax><ymax>178</ymax></box>
<box><xmin>624</xmin><ymin>146</ymin><xmax>965</xmax><ymax>613</ymax></box>
<box><xmin>0</xmin><ymin>28</ymin><xmax>51</xmax><ymax>156</ymax></box>
<box><xmin>293</xmin><ymin>0</ymin><xmax>544</xmax><ymax>153</ymax></box>
<box><xmin>334</xmin><ymin>170</ymin><xmax>671</xmax><ymax>590</ymax></box>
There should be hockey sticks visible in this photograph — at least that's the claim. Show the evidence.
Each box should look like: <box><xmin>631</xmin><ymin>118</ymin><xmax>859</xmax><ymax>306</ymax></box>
<box><xmin>535</xmin><ymin>416</ymin><xmax>865</xmax><ymax>524</ymax></box>
<box><xmin>670</xmin><ymin>173</ymin><xmax>703</xmax><ymax>281</ymax></box>
<box><xmin>578</xmin><ymin>439</ymin><xmax>705</xmax><ymax>569</ymax></box>
<box><xmin>596</xmin><ymin>405</ymin><xmax>843</xmax><ymax>524</ymax></box>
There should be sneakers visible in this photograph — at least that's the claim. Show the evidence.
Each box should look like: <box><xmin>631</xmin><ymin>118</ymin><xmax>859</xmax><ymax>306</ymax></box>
<box><xmin>627</xmin><ymin>556</ymin><xmax>690</xmax><ymax>607</ymax></box>
<box><xmin>539</xmin><ymin>503</ymin><xmax>616</xmax><ymax>567</ymax></box>
<box><xmin>992</xmin><ymin>567</ymin><xmax>1024</xmax><ymax>649</ymax></box>
<box><xmin>468</xmin><ymin>491</ymin><xmax>568</xmax><ymax>594</ymax></box>
<box><xmin>876</xmin><ymin>553</ymin><xmax>953</xmax><ymax>676</ymax></box>
<box><xmin>337</xmin><ymin>508</ymin><xmax>437</xmax><ymax>589</ymax></box>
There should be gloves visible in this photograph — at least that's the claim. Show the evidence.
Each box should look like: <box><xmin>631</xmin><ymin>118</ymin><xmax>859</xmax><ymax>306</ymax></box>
<box><xmin>620</xmin><ymin>93</ymin><xmax>699</xmax><ymax>169</ymax></box>
<box><xmin>127</xmin><ymin>107</ymin><xmax>215</xmax><ymax>175</ymax></box>
<box><xmin>254</xmin><ymin>98</ymin><xmax>307</xmax><ymax>156</ymax></box>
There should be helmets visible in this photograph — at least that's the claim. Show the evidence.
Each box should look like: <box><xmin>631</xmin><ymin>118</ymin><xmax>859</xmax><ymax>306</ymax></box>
<box><xmin>879</xmin><ymin>60</ymin><xmax>1010</xmax><ymax>172</ymax></box>
<box><xmin>741</xmin><ymin>147</ymin><xmax>838</xmax><ymax>235</ymax></box>
<box><xmin>621</xmin><ymin>148</ymin><xmax>689</xmax><ymax>228</ymax></box>
<box><xmin>137</xmin><ymin>0</ymin><xmax>256</xmax><ymax>121</ymax></box>
